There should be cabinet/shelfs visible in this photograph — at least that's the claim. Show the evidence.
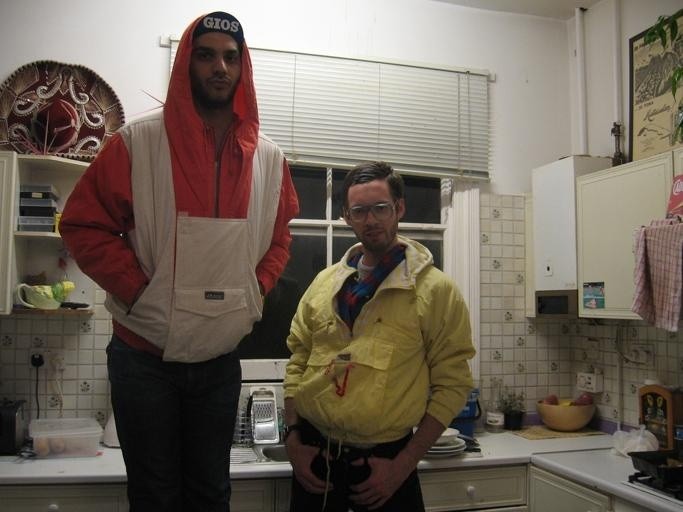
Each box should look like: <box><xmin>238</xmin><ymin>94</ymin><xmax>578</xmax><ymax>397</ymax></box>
<box><xmin>0</xmin><ymin>149</ymin><xmax>98</xmax><ymax>319</ymax></box>
<box><xmin>0</xmin><ymin>465</ymin><xmax>664</xmax><ymax>512</ymax></box>
<box><xmin>524</xmin><ymin>154</ymin><xmax>668</xmax><ymax>324</ymax></box>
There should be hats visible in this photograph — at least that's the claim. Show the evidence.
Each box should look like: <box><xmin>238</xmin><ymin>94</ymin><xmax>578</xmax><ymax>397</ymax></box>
<box><xmin>192</xmin><ymin>11</ymin><xmax>245</xmax><ymax>50</ymax></box>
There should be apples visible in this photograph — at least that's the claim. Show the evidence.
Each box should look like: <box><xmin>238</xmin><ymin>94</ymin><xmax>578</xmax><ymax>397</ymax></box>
<box><xmin>538</xmin><ymin>394</ymin><xmax>593</xmax><ymax>405</ymax></box>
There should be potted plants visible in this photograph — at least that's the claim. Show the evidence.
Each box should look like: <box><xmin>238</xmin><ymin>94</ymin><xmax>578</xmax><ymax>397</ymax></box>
<box><xmin>496</xmin><ymin>381</ymin><xmax>529</xmax><ymax>432</ymax></box>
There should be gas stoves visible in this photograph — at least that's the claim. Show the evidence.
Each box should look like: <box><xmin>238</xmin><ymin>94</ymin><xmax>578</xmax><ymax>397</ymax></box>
<box><xmin>628</xmin><ymin>468</ymin><xmax>683</xmax><ymax>504</ymax></box>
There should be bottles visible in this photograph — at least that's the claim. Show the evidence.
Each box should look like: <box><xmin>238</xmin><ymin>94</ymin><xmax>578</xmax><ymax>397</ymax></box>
<box><xmin>485</xmin><ymin>383</ymin><xmax>506</xmax><ymax>433</ymax></box>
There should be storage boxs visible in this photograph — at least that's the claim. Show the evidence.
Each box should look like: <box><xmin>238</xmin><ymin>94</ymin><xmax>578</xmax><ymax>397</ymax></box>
<box><xmin>27</xmin><ymin>418</ymin><xmax>103</xmax><ymax>458</ymax></box>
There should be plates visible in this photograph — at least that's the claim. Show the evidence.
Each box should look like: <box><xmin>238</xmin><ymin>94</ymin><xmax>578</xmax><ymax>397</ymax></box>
<box><xmin>412</xmin><ymin>438</ymin><xmax>467</xmax><ymax>458</ymax></box>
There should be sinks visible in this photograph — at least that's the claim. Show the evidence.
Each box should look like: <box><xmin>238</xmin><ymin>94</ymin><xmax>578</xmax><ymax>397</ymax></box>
<box><xmin>254</xmin><ymin>442</ymin><xmax>292</xmax><ymax>465</ymax></box>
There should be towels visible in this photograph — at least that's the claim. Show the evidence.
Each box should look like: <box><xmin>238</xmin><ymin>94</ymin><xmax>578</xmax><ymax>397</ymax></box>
<box><xmin>628</xmin><ymin>218</ymin><xmax>682</xmax><ymax>333</ymax></box>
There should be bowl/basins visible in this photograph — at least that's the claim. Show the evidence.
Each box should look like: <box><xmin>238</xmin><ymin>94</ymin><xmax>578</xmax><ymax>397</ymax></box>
<box><xmin>536</xmin><ymin>398</ymin><xmax>598</xmax><ymax>432</ymax></box>
<box><xmin>413</xmin><ymin>428</ymin><xmax>460</xmax><ymax>445</ymax></box>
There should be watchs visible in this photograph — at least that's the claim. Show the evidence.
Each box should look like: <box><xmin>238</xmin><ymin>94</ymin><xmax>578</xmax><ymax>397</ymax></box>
<box><xmin>279</xmin><ymin>422</ymin><xmax>298</xmax><ymax>443</ymax></box>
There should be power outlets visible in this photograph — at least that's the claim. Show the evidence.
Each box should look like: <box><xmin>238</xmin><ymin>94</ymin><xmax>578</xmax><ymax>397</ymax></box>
<box><xmin>627</xmin><ymin>344</ymin><xmax>655</xmax><ymax>366</ymax></box>
<box><xmin>27</xmin><ymin>348</ymin><xmax>69</xmax><ymax>371</ymax></box>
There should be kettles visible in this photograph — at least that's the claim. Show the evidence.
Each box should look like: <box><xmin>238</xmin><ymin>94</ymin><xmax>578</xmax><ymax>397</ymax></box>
<box><xmin>0</xmin><ymin>396</ymin><xmax>26</xmax><ymax>455</ymax></box>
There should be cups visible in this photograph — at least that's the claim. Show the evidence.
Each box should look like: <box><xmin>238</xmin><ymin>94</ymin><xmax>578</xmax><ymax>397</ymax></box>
<box><xmin>17</xmin><ymin>282</ymin><xmax>64</xmax><ymax>310</ymax></box>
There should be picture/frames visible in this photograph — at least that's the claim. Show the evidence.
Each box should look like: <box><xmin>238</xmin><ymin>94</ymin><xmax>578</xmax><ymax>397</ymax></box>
<box><xmin>627</xmin><ymin>9</ymin><xmax>683</xmax><ymax>163</ymax></box>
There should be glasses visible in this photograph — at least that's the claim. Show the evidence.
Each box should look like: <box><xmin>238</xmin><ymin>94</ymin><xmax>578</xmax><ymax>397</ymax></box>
<box><xmin>343</xmin><ymin>200</ymin><xmax>400</xmax><ymax>226</ymax></box>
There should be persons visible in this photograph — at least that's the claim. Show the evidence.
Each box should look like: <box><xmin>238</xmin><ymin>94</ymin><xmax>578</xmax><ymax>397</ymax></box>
<box><xmin>278</xmin><ymin>159</ymin><xmax>476</xmax><ymax>511</ymax></box>
<box><xmin>58</xmin><ymin>11</ymin><xmax>301</xmax><ymax>511</ymax></box>
